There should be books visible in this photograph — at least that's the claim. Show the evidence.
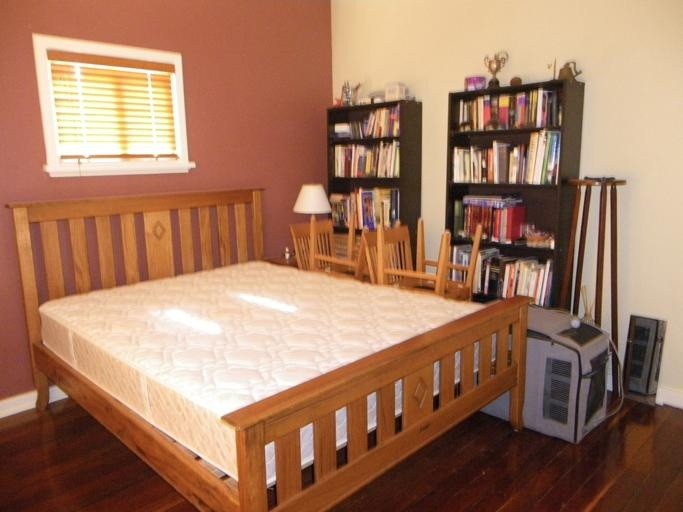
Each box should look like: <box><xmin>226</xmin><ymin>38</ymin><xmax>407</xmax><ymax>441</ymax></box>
<box><xmin>451</xmin><ymin>88</ymin><xmax>564</xmax><ymax>308</ymax></box>
<box><xmin>332</xmin><ymin>106</ymin><xmax>399</xmax><ymax>231</ymax></box>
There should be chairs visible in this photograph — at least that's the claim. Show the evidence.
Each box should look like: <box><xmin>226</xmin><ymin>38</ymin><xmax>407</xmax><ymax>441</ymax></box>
<box><xmin>286</xmin><ymin>221</ymin><xmax>483</xmax><ymax>303</ymax></box>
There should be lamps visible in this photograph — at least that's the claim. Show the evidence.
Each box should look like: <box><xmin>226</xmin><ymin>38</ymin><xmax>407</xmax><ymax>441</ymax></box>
<box><xmin>292</xmin><ymin>185</ymin><xmax>333</xmax><ymax>273</ymax></box>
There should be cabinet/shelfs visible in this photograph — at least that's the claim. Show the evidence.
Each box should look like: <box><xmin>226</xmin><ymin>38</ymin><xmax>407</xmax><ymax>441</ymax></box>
<box><xmin>443</xmin><ymin>79</ymin><xmax>586</xmax><ymax>314</ymax></box>
<box><xmin>326</xmin><ymin>100</ymin><xmax>422</xmax><ymax>275</ymax></box>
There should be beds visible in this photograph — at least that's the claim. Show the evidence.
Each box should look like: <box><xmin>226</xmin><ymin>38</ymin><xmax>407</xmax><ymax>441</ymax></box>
<box><xmin>4</xmin><ymin>189</ymin><xmax>532</xmax><ymax>512</ymax></box>
